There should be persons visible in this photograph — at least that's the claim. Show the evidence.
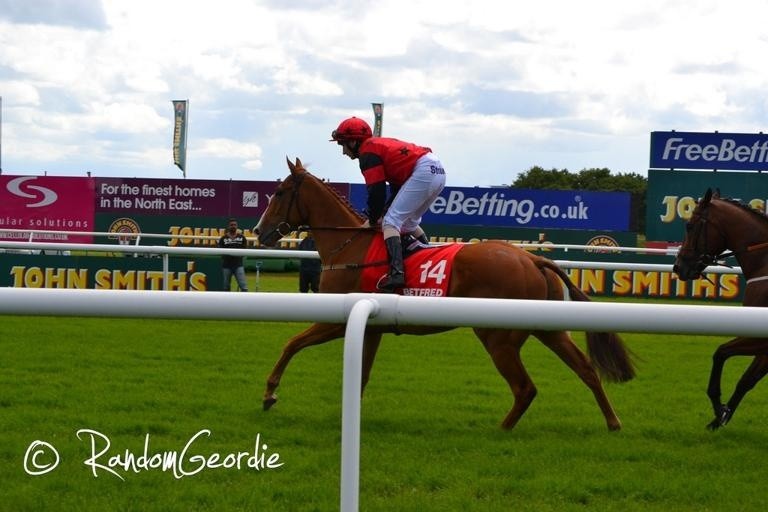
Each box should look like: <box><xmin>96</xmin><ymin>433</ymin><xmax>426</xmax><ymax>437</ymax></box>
<box><xmin>219</xmin><ymin>220</ymin><xmax>248</xmax><ymax>292</ymax></box>
<box><xmin>297</xmin><ymin>231</ymin><xmax>323</xmax><ymax>293</ymax></box>
<box><xmin>328</xmin><ymin>116</ymin><xmax>446</xmax><ymax>290</ymax></box>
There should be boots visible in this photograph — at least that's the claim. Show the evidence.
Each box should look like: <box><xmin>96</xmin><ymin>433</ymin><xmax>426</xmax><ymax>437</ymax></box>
<box><xmin>378</xmin><ymin>236</ymin><xmax>404</xmax><ymax>288</ymax></box>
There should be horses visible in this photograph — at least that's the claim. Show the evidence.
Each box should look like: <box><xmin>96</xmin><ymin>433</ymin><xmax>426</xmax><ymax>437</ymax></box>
<box><xmin>670</xmin><ymin>185</ymin><xmax>768</xmax><ymax>432</ymax></box>
<box><xmin>252</xmin><ymin>155</ymin><xmax>645</xmax><ymax>433</ymax></box>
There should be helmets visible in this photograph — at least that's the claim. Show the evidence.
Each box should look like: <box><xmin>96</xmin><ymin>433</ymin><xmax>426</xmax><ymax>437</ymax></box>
<box><xmin>329</xmin><ymin>116</ymin><xmax>371</xmax><ymax>140</ymax></box>
